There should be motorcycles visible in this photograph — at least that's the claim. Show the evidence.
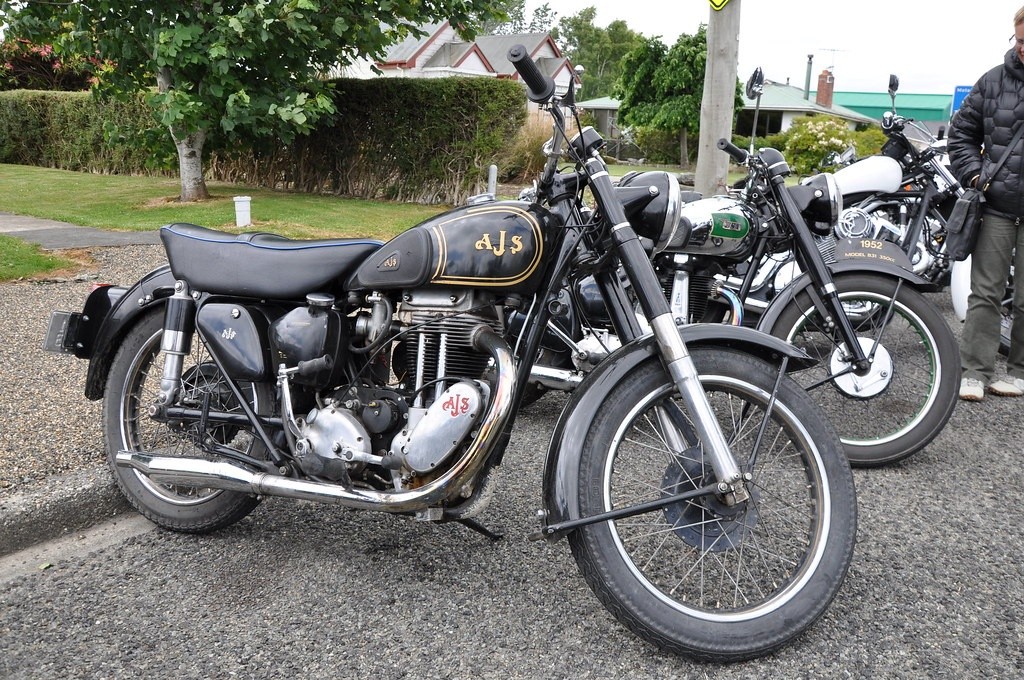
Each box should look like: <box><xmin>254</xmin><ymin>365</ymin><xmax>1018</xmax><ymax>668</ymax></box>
<box><xmin>514</xmin><ymin>73</ymin><xmax>1016</xmax><ymax>470</ymax></box>
<box><xmin>39</xmin><ymin>43</ymin><xmax>860</xmax><ymax>667</ymax></box>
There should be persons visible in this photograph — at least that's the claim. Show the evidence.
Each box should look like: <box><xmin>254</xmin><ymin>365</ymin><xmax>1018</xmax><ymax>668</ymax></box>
<box><xmin>947</xmin><ymin>6</ymin><xmax>1024</xmax><ymax>400</ymax></box>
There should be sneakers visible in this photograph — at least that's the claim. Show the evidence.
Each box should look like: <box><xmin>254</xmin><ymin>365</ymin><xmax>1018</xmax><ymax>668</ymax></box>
<box><xmin>959</xmin><ymin>378</ymin><xmax>984</xmax><ymax>401</ymax></box>
<box><xmin>988</xmin><ymin>374</ymin><xmax>1024</xmax><ymax>397</ymax></box>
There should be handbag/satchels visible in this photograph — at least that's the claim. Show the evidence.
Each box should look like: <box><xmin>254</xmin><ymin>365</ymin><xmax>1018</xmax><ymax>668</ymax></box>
<box><xmin>944</xmin><ymin>188</ymin><xmax>986</xmax><ymax>262</ymax></box>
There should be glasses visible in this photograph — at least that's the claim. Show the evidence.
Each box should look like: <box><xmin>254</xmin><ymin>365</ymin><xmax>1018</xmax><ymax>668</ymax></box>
<box><xmin>1009</xmin><ymin>34</ymin><xmax>1024</xmax><ymax>48</ymax></box>
